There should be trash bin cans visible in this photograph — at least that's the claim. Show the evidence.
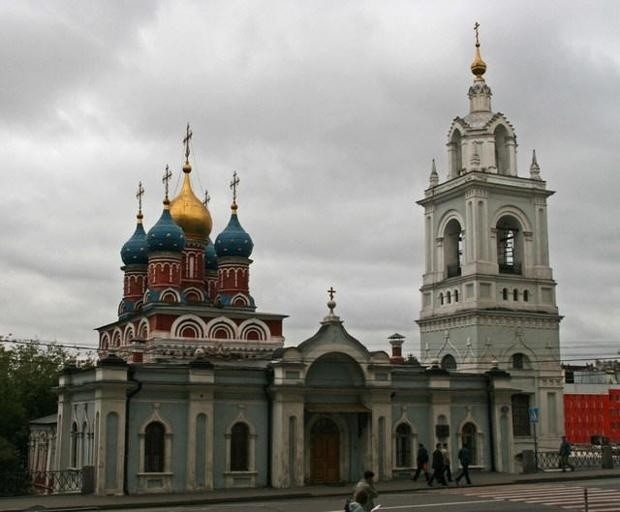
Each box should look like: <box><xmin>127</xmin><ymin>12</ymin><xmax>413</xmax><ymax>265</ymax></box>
<box><xmin>601</xmin><ymin>445</ymin><xmax>614</xmax><ymax>469</ymax></box>
<box><xmin>523</xmin><ymin>450</ymin><xmax>535</xmax><ymax>473</ymax></box>
<box><xmin>82</xmin><ymin>465</ymin><xmax>95</xmax><ymax>495</ymax></box>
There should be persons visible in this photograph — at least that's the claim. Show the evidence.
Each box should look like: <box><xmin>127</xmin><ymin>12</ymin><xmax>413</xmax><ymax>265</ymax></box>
<box><xmin>428</xmin><ymin>443</ymin><xmax>448</xmax><ymax>487</ymax></box>
<box><xmin>559</xmin><ymin>436</ymin><xmax>575</xmax><ymax>472</ymax></box>
<box><xmin>352</xmin><ymin>471</ymin><xmax>378</xmax><ymax>511</ymax></box>
<box><xmin>348</xmin><ymin>488</ymin><xmax>372</xmax><ymax>512</ymax></box>
<box><xmin>442</xmin><ymin>443</ymin><xmax>453</xmax><ymax>482</ymax></box>
<box><xmin>411</xmin><ymin>443</ymin><xmax>428</xmax><ymax>482</ymax></box>
<box><xmin>456</xmin><ymin>442</ymin><xmax>473</xmax><ymax>485</ymax></box>
